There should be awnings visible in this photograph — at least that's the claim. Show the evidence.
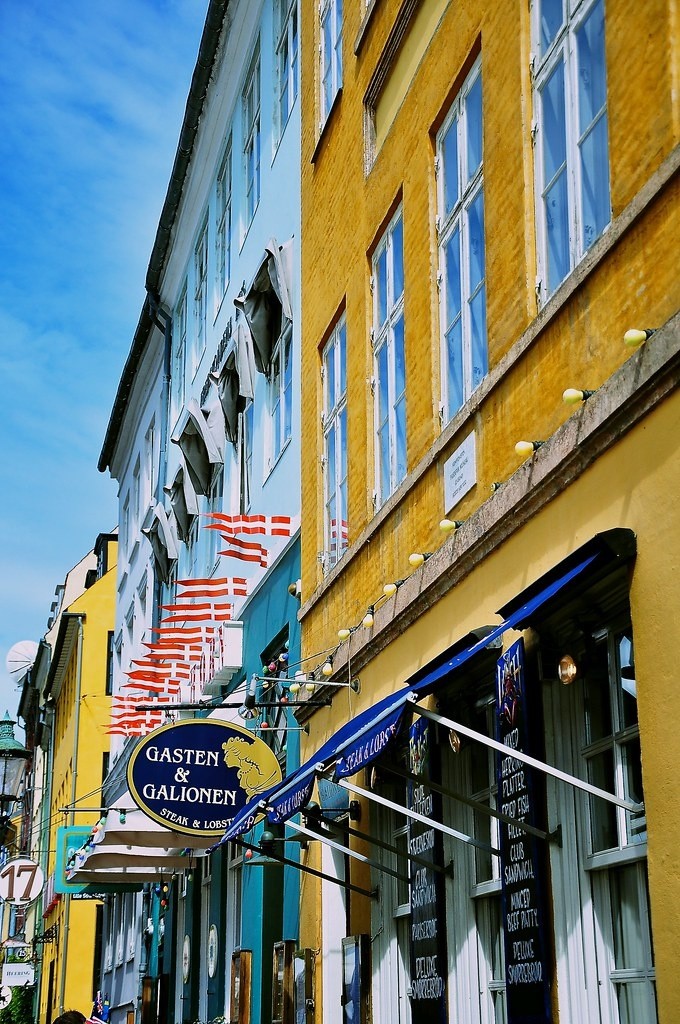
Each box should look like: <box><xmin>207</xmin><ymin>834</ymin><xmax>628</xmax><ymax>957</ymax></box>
<box><xmin>207</xmin><ymin>556</ymin><xmax>640</xmax><ymax>896</ymax></box>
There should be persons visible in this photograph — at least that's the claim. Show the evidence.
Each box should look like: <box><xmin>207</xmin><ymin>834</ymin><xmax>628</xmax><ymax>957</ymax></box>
<box><xmin>52</xmin><ymin>1011</ymin><xmax>86</xmax><ymax>1024</ymax></box>
